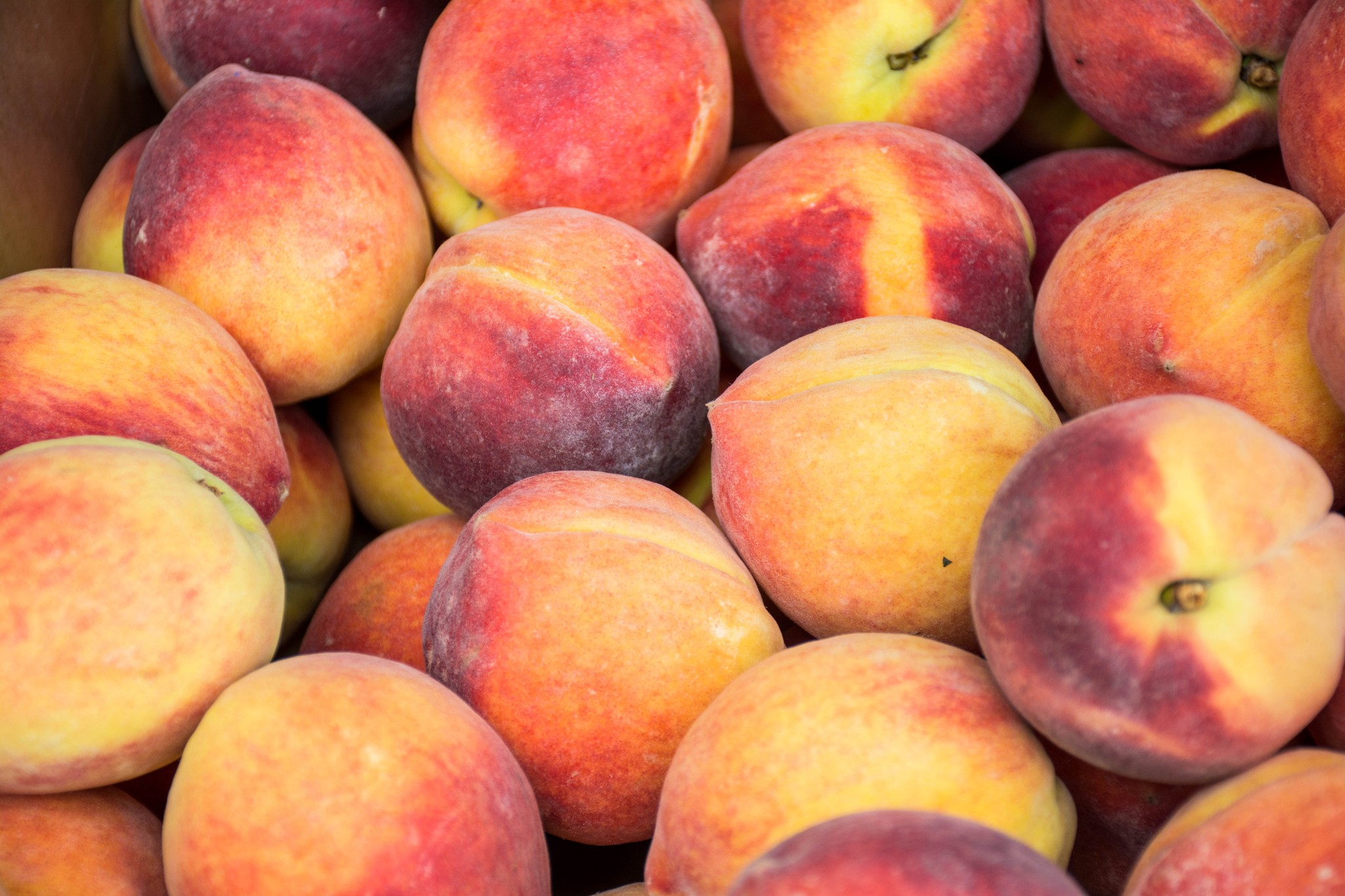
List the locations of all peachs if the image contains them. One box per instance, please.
[0,0,1345,896]
